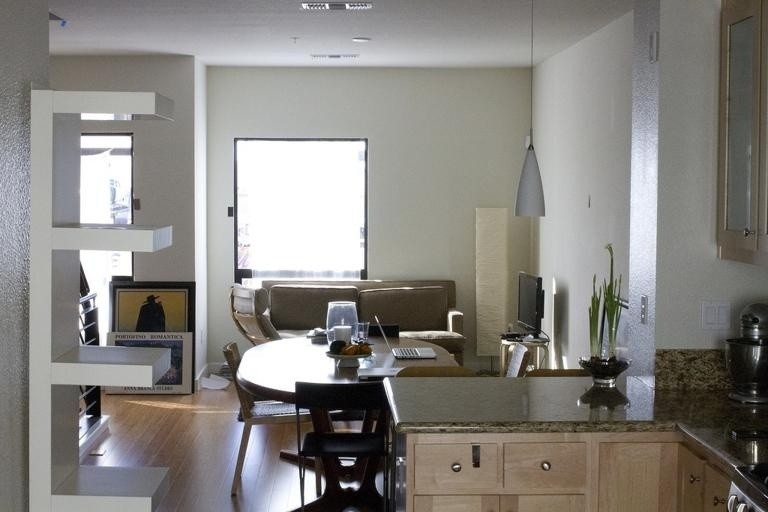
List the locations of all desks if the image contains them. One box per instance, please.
[498,338,550,376]
[236,334,458,495]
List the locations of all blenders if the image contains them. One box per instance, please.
[724,300,768,402]
[726,403,768,467]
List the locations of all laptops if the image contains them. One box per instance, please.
[375,315,438,360]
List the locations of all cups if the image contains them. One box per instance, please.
[356,321,371,340]
[326,301,360,348]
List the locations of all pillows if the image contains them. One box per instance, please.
[358,286,447,331]
[270,285,359,330]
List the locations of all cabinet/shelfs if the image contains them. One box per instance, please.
[598,437,679,512]
[27,89,173,512]
[679,443,731,512]
[716,0,768,266]
[411,436,588,511]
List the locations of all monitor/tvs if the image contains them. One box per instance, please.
[517,272,545,335]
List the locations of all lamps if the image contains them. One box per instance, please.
[515,0,546,217]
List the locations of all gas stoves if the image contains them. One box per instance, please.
[722,461,768,512]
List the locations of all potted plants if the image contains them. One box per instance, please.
[576,242,631,388]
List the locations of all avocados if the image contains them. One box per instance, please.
[330,340,346,354]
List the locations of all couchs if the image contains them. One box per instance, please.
[261,280,467,365]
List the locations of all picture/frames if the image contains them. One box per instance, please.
[107,331,193,394]
[109,281,195,395]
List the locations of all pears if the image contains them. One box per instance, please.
[342,344,371,355]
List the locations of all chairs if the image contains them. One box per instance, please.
[293,380,397,511]
[370,366,476,502]
[223,341,326,497]
[228,284,281,346]
[503,344,530,377]
